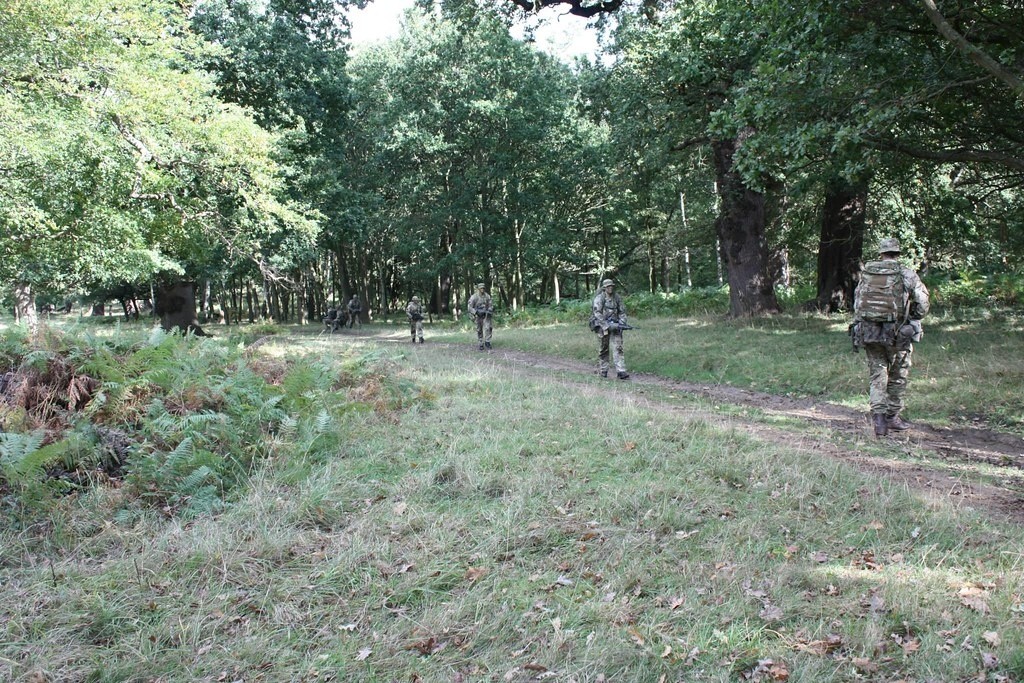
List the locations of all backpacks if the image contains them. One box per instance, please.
[857,262,905,323]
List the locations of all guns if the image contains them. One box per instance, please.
[413,314,424,321]
[475,306,492,320]
[608,318,634,335]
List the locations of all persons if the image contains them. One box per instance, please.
[322,294,363,332]
[592,278,630,380]
[405,296,424,344]
[467,283,494,350]
[847,238,930,440]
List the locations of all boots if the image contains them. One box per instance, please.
[885,414,912,431]
[872,415,889,438]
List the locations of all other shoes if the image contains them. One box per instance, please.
[485,342,491,349]
[480,346,484,350]
[412,338,415,342]
[419,338,424,343]
[617,372,629,379]
[601,371,608,377]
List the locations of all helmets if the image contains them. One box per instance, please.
[411,296,419,300]
[602,279,615,287]
[478,283,485,288]
[879,239,901,253]
[353,295,357,298]
